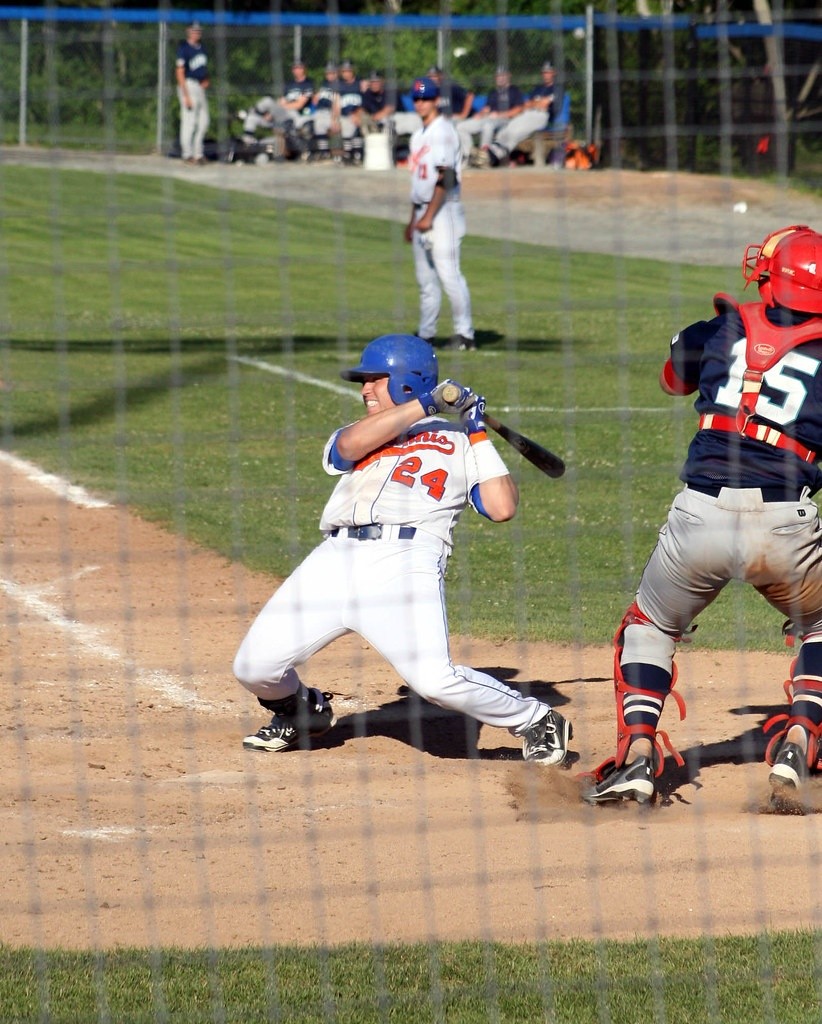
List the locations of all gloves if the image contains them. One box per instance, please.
[456,390,489,437]
[416,379,477,417]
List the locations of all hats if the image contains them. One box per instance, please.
[290,61,305,68]
[541,61,551,71]
[370,71,384,80]
[340,62,352,70]
[324,64,337,71]
[187,23,201,29]
[426,66,439,76]
[493,66,510,73]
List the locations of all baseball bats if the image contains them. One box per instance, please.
[442,384,566,479]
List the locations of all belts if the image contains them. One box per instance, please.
[686,482,801,503]
[414,202,427,208]
[331,524,415,540]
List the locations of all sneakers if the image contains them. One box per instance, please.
[767,742,808,805]
[578,755,654,806]
[241,688,336,751]
[520,710,573,768]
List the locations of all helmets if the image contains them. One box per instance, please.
[741,226,822,312]
[341,333,438,404]
[408,78,440,102]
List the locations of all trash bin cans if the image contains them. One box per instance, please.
[361,119,397,170]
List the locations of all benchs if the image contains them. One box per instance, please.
[264,88,574,169]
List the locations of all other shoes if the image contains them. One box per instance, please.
[443,335,477,355]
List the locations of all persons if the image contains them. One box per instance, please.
[176,22,208,163]
[572,227,822,815]
[404,76,476,352]
[233,335,571,766]
[227,62,564,170]
